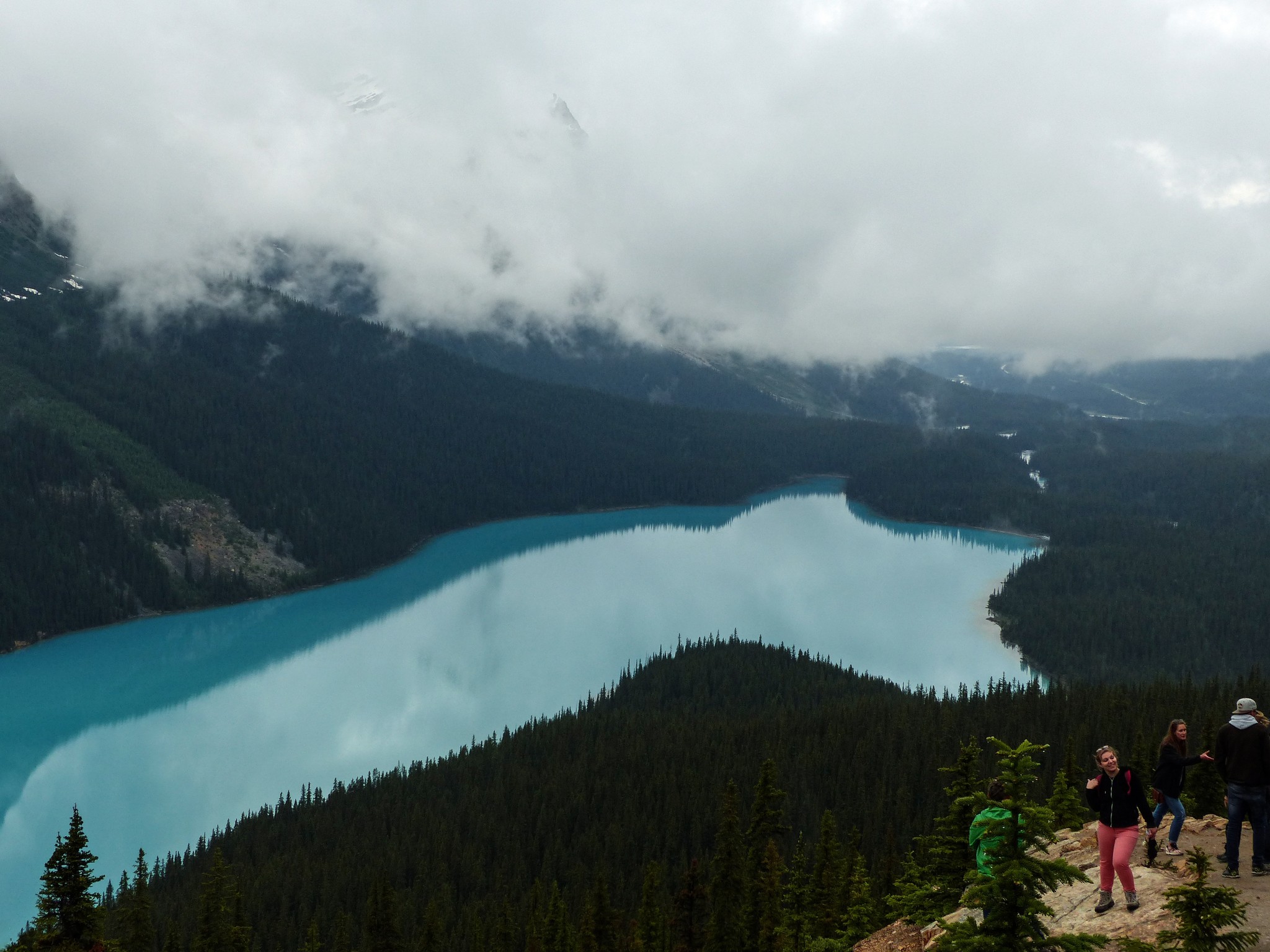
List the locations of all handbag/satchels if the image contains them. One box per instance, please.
[1152,788,1164,805]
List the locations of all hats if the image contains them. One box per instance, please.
[1232,698,1257,714]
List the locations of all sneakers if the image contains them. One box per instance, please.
[1251,860,1270,876]
[1124,889,1139,910]
[1222,865,1240,878]
[1216,852,1240,864]
[1143,838,1164,852]
[1166,843,1184,855]
[1094,888,1114,913]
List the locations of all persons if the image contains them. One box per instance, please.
[1086,745,1157,913]
[1216,711,1270,869]
[1142,719,1215,855]
[1214,698,1270,877]
[969,781,1025,921]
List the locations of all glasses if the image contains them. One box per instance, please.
[1096,745,1109,755]
